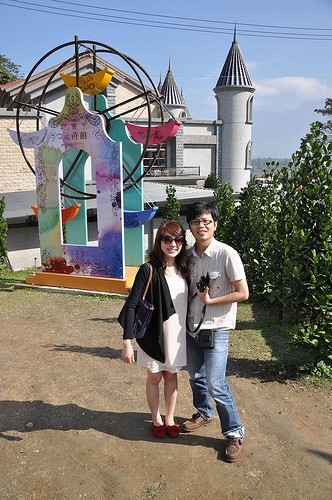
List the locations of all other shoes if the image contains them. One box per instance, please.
[152,420,166,438]
[163,417,179,437]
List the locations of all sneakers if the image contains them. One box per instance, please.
[183,412,212,432]
[223,426,245,463]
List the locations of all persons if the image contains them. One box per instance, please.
[121,221,191,439]
[184,201,249,462]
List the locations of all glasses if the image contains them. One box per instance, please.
[190,219,212,225]
[161,235,185,245]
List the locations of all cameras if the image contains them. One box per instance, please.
[196,271,219,293]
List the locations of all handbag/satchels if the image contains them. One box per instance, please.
[117,261,154,339]
